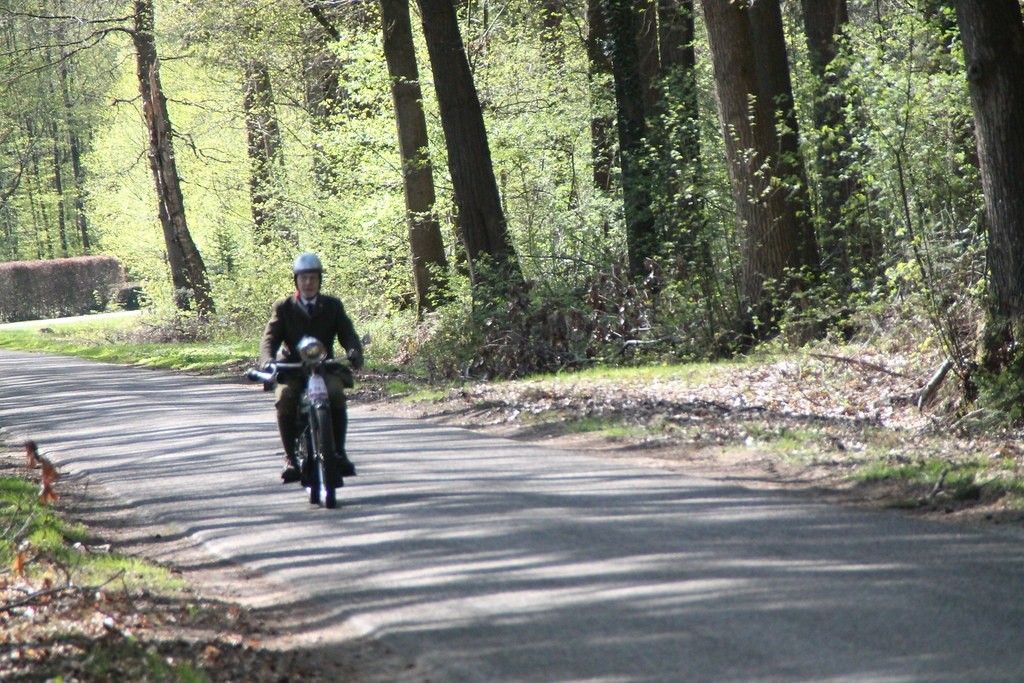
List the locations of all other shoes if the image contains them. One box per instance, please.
[336,453,356,476]
[281,458,300,478]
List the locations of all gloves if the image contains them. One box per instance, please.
[260,359,277,373]
[348,349,362,369]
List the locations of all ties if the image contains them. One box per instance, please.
[306,302,314,314]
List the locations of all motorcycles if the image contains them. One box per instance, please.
[246,333,372,509]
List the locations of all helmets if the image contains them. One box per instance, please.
[293,253,322,275]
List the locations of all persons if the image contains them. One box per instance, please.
[260,254,363,480]
